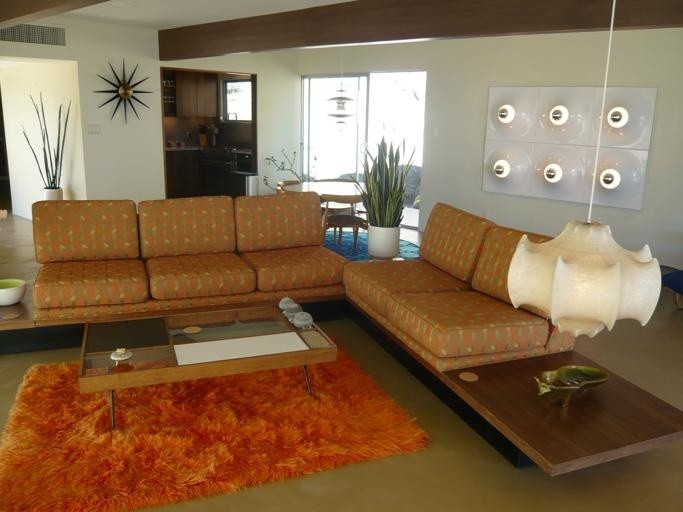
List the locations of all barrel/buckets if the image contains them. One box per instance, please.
[198,134,206,145]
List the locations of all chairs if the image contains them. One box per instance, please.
[274,178,367,255]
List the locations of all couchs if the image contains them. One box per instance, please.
[30,190,351,327]
[336,198,681,479]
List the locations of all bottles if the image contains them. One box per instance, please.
[278,296,312,329]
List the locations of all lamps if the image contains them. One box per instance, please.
[328,44,352,123]
[504,0,662,338]
[480,86,658,212]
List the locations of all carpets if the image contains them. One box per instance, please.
[1,334,431,512]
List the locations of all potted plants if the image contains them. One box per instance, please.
[353,134,416,257]
[21,87,70,200]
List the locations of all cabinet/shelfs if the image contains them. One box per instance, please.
[174,71,218,119]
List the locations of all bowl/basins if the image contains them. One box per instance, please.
[0,279,26,306]
